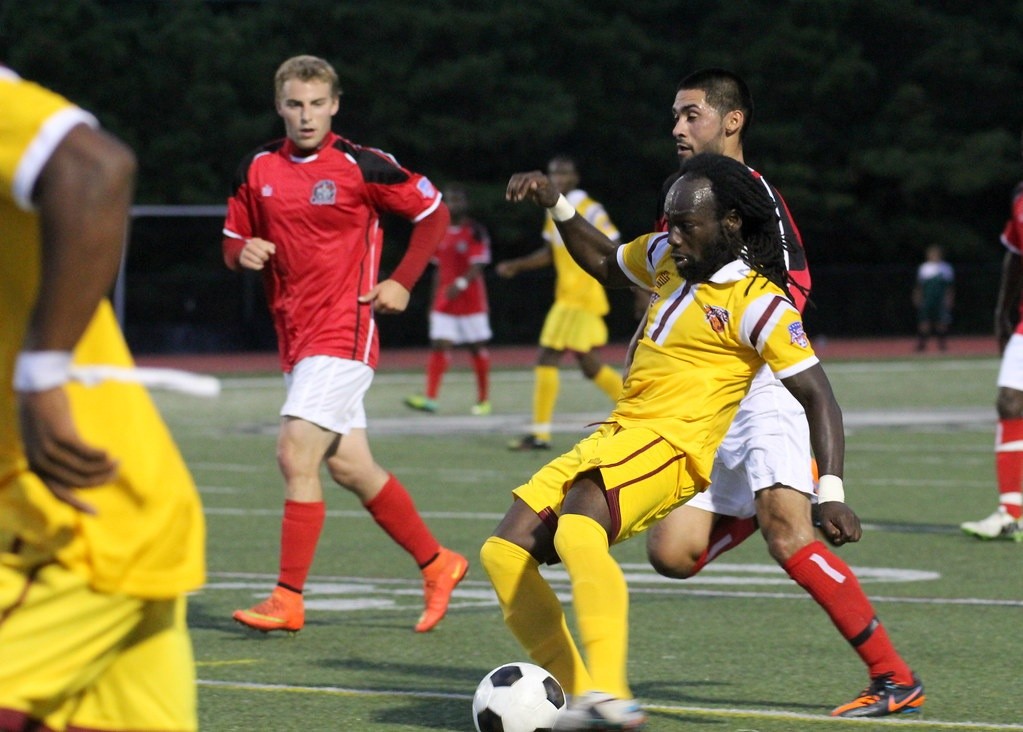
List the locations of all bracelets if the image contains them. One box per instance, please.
[455,277,468,290]
[11,349,71,392]
[818,474,845,505]
[549,193,575,221]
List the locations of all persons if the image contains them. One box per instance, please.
[913,246,955,351]
[222,58,469,633]
[647,69,925,718]
[0,298,208,732]
[0,64,135,515]
[402,188,493,416]
[960,189,1023,540]
[480,147,862,732]
[497,153,622,449]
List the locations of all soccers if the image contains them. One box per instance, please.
[472,662,567,731]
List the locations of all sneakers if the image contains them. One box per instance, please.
[555,693,645,730]
[831,673,925,718]
[405,394,438,411]
[472,402,490,415]
[508,436,549,450]
[234,595,304,633]
[959,507,1022,542]
[414,550,468,631]
